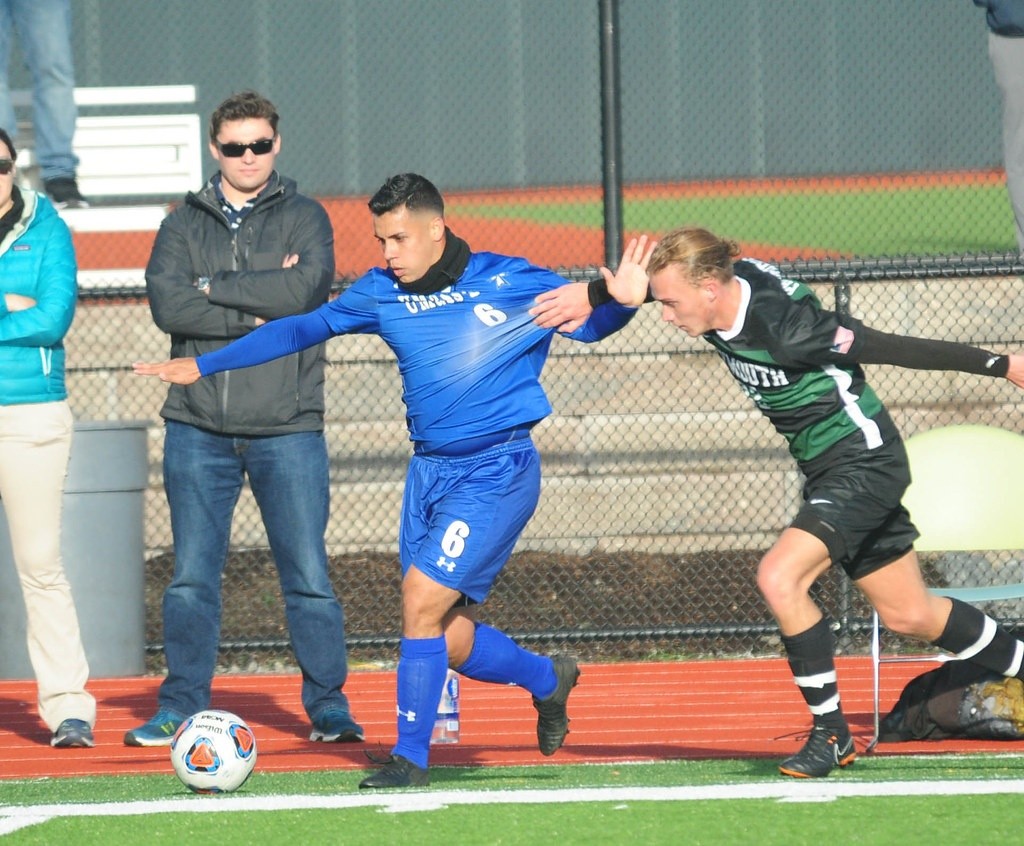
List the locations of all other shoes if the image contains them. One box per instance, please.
[46,177,89,210]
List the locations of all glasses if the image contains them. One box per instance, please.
[0,159,13,174]
[215,136,275,157]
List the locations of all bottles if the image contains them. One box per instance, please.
[430,669,460,745]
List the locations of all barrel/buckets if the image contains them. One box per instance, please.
[0,421,155,678]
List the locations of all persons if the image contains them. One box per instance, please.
[0,1,93,211]
[531,228,1023,779]
[133,175,659,791]
[971,1,1024,255]
[0,129,96,748]
[124,95,367,748]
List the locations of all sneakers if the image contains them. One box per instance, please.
[124,706,189,748]
[773,719,856,777]
[530,654,580,756]
[51,719,94,747]
[358,741,431,789]
[310,711,365,742]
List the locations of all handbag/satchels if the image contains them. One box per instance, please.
[879,662,1024,742]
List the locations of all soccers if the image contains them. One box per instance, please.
[169,710,257,794]
